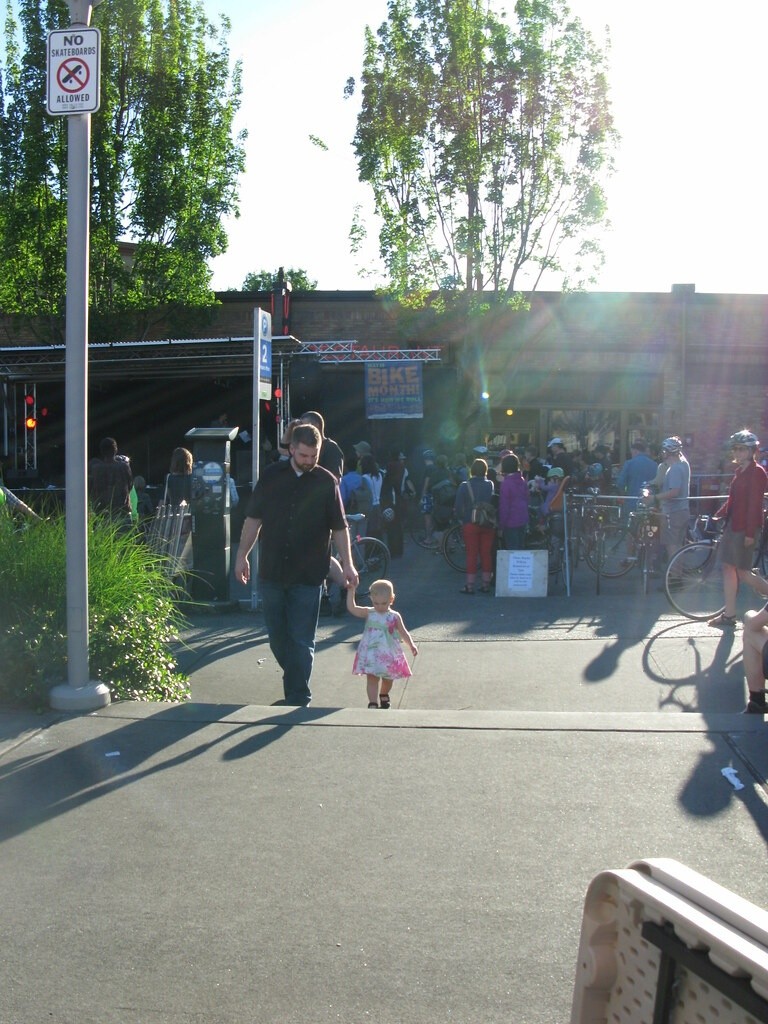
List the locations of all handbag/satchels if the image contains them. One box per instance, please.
[380,503,398,522]
[429,504,450,535]
[431,479,457,505]
[403,488,413,499]
[472,501,498,530]
[347,478,371,519]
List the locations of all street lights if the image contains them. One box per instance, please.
[271,264,293,458]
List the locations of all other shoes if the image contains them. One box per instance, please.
[739,701,768,714]
[478,586,490,594]
[657,577,685,593]
[379,693,390,709]
[536,524,547,532]
[620,554,638,567]
[368,702,378,709]
[459,585,474,594]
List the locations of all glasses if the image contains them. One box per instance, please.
[731,445,747,452]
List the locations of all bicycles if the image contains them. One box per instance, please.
[664,515,768,619]
[409,507,590,576]
[585,485,723,578]
[322,513,391,603]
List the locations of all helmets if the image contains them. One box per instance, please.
[473,446,488,456]
[588,463,603,477]
[728,430,760,447]
[661,436,683,454]
[497,449,514,458]
[547,467,565,480]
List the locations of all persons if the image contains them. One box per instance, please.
[269,441,416,572]
[0,486,43,524]
[740,602,768,714]
[88,437,239,602]
[707,429,768,626]
[235,411,418,709]
[418,437,612,594]
[616,436,690,593]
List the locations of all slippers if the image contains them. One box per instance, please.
[417,535,432,545]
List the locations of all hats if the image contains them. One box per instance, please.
[422,447,434,459]
[399,452,407,460]
[547,437,563,448]
[352,440,372,457]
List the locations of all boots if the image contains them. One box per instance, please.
[318,592,334,616]
[335,589,357,617]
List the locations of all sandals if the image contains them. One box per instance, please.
[706,612,737,625]
